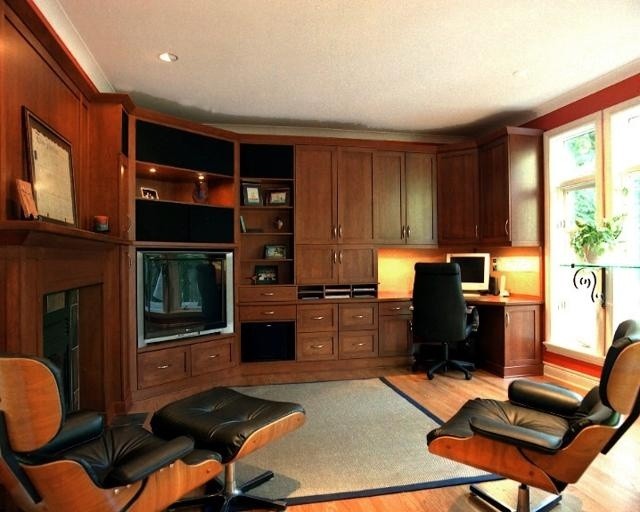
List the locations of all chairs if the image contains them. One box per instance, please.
[412,262,480,379]
[0,352,226,512]
[426,319,640,512]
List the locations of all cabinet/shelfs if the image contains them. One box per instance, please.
[0,0,545,429]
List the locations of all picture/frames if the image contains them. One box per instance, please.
[255,265,279,284]
[265,187,291,205]
[241,182,264,206]
[21,105,79,228]
[140,186,160,200]
[264,244,287,260]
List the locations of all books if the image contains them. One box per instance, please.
[14,177,40,220]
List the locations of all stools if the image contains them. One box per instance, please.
[149,386,305,512]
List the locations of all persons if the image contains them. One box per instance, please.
[256,270,274,280]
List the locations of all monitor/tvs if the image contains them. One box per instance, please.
[136,251,234,349]
[446,253,491,297]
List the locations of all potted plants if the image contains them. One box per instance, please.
[567,210,628,263]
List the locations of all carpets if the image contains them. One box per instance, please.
[143,377,510,505]
